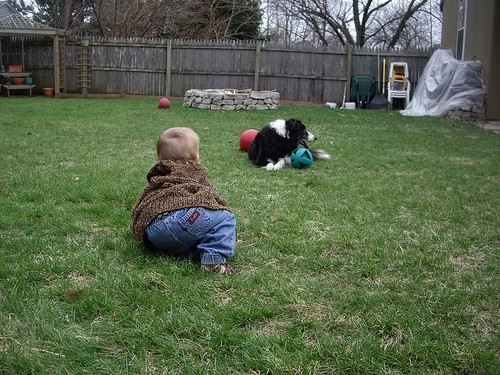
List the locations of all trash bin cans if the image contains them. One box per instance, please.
[351,74,375,108]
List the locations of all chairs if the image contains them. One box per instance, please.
[387,62,411,110]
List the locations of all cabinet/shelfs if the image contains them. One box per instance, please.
[0,73,37,97]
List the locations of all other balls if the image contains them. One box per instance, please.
[239,128,259,150]
[159,97,171,108]
[290,147,314,169]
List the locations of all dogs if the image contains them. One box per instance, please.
[248,118,331,171]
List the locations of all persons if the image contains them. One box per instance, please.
[131,127,238,275]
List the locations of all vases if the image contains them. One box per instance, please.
[42,88,54,97]
[26,78,32,84]
[12,78,25,85]
[9,66,22,73]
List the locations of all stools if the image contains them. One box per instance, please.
[392,75,406,90]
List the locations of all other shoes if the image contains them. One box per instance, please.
[200,263,236,274]
